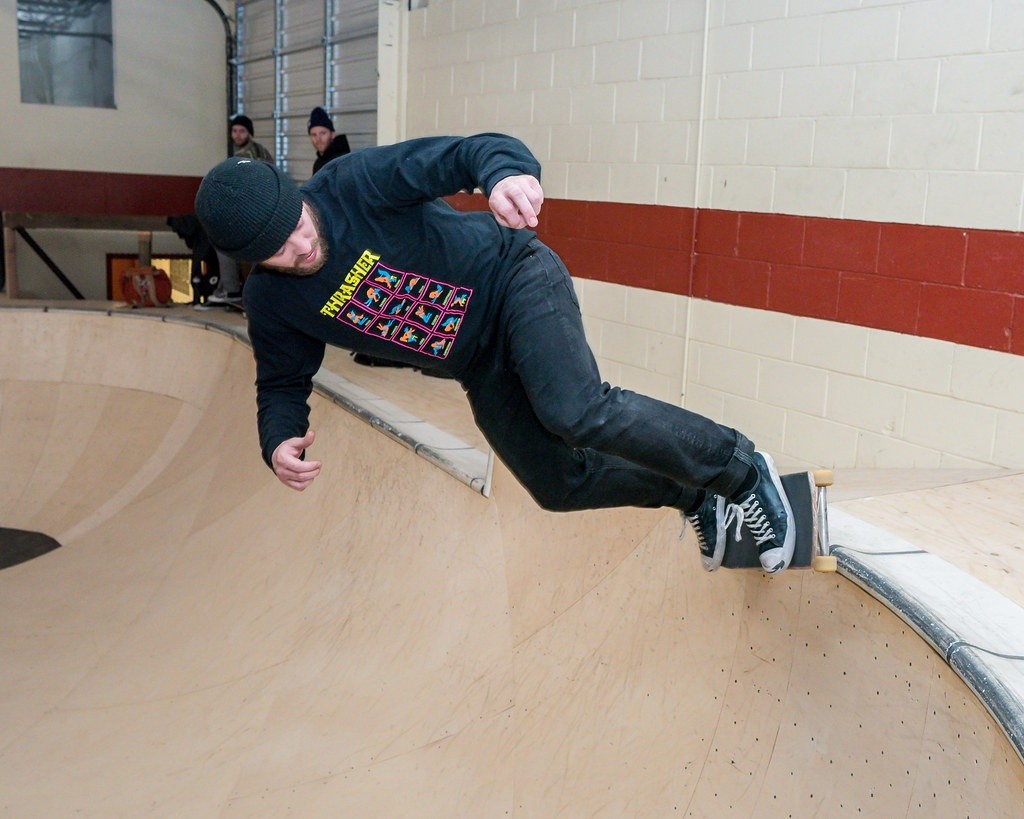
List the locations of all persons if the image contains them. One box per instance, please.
[194,132,795,572]
[194,116,273,310]
[307,106,351,176]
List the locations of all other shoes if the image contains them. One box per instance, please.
[208,289,243,302]
[194,299,227,311]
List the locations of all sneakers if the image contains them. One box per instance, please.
[720,452,795,575]
[681,493,727,572]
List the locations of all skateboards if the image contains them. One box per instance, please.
[720,471,838,571]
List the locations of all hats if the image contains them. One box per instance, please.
[195,156,302,264]
[231,116,254,138]
[307,107,335,134]
